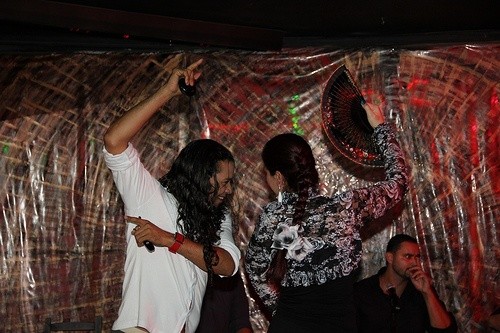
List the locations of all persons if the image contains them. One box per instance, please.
[102,58,241,333]
[243,102,408,333]
[357,234,458,333]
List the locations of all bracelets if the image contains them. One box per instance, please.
[169,232,184,254]
